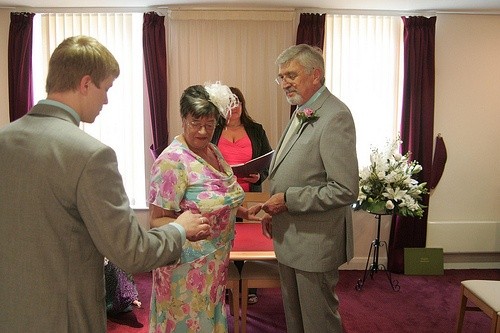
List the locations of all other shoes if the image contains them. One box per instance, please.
[247,293,258,304]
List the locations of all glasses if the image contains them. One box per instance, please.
[274,71,305,85]
[184,117,219,130]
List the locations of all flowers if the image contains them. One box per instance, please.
[353,130,431,221]
[295,107,320,135]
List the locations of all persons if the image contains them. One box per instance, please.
[261,43,359,333]
[149,84,265,332]
[211,86,274,304]
[0,35,211,332]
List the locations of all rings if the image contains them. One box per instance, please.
[200,217,204,223]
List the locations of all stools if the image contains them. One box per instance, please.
[225,261,281,333]
[455,279,500,333]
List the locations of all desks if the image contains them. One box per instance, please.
[229,222,276,260]
